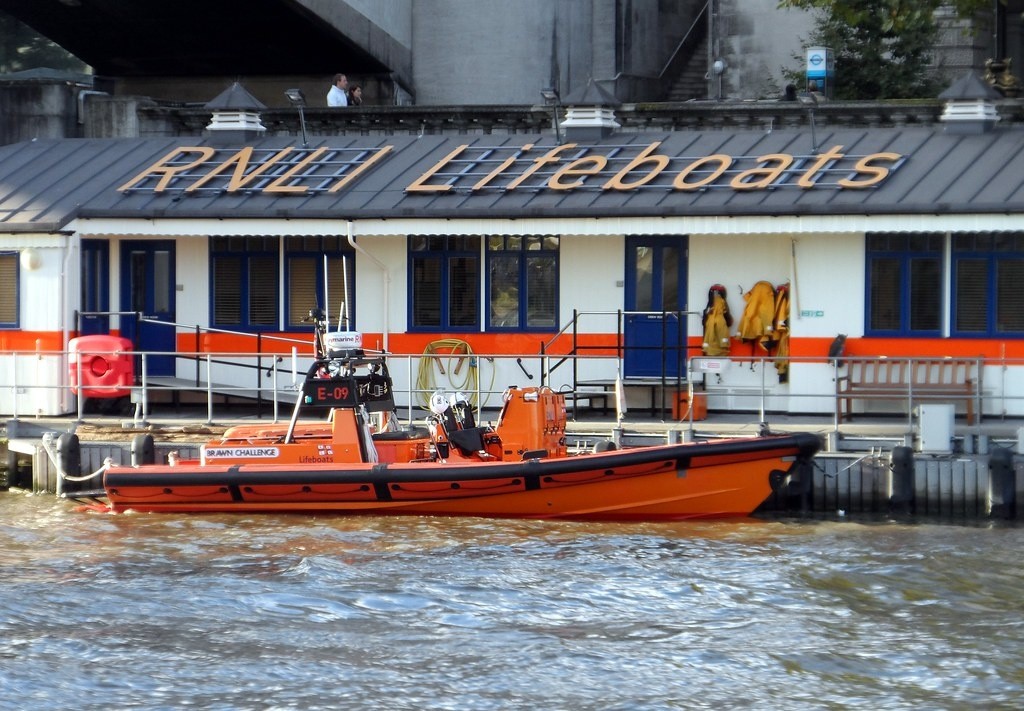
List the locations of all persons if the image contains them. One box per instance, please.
[326,74,348,106]
[345,84,362,106]
[803,80,829,102]
[779,84,798,101]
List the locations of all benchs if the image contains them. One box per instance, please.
[837,354,977,425]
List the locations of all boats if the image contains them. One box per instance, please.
[104,249,827,524]
